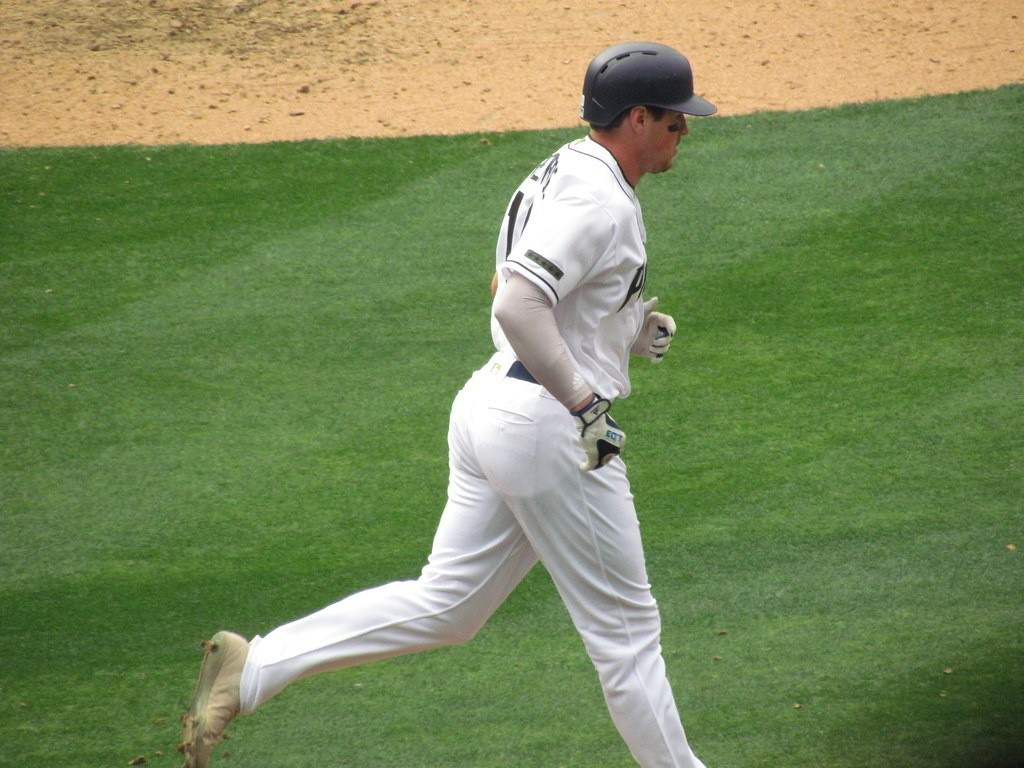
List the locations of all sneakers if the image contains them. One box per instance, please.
[176,630,249,768]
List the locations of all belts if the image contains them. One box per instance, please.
[506,361,542,386]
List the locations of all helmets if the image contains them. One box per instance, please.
[579,41,717,127]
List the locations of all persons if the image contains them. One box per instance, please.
[183,41,718,768]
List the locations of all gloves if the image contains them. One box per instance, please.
[630,297,676,363]
[570,394,625,471]
